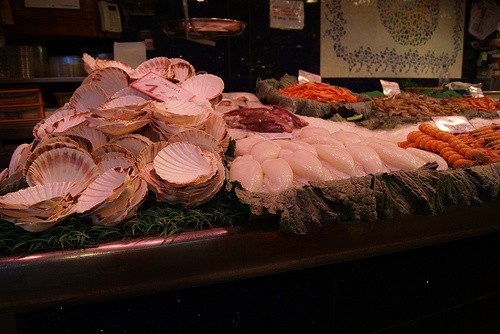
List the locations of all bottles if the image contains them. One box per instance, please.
[438,65,450,87]
[476,52,490,92]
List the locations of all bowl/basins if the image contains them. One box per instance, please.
[54,92,74,107]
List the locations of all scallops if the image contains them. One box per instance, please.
[0,55,232,233]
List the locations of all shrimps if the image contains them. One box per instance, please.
[397,123,500,170]
[372,90,500,119]
[279,81,358,105]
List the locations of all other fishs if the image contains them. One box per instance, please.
[222,105,310,134]
